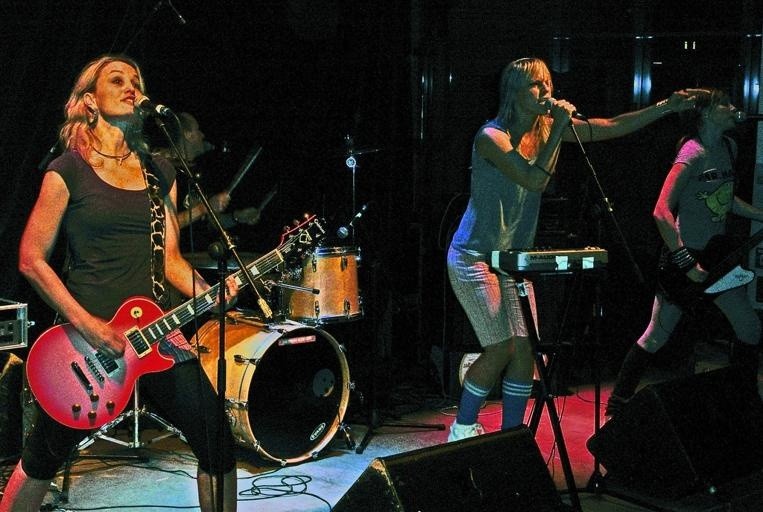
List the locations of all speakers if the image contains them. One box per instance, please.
[585,363,763,503]
[0,349,25,468]
[329,423,567,512]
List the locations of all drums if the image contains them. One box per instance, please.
[274,242,364,328]
[186,309,351,465]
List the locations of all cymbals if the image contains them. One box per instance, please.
[180,250,262,271]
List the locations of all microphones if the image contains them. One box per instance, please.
[734,111,763,123]
[545,98,589,122]
[134,94,171,117]
[336,202,371,239]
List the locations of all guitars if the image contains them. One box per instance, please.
[26,214,327,431]
[658,229,763,318]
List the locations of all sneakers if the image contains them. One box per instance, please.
[448,419,485,443]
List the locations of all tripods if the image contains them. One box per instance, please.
[556,288,676,512]
[76,376,188,452]
[356,259,446,454]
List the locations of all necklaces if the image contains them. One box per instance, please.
[91,146,134,166]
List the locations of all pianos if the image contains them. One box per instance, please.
[486,244,608,274]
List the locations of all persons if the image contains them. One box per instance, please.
[603,89,762,420]
[148,110,264,313]
[446,57,711,443]
[0,54,240,512]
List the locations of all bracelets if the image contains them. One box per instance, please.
[670,244,696,276]
[199,203,205,214]
[230,210,240,225]
[655,98,672,117]
[535,164,554,179]
